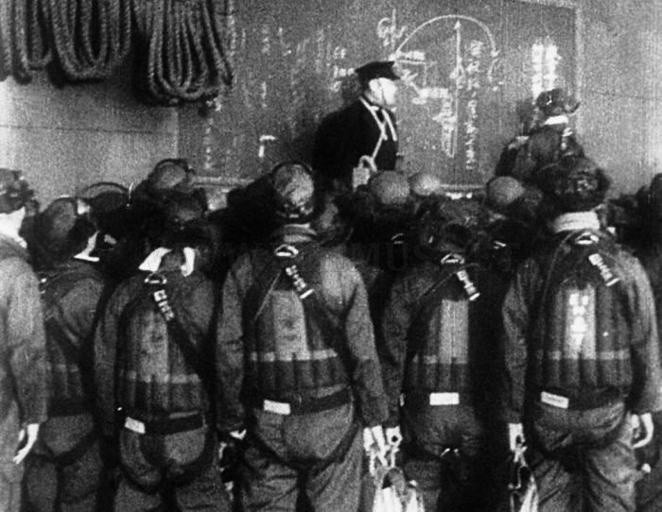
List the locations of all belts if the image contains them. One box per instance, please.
[45,399,90,417]
[408,390,476,408]
[119,413,205,435]
[247,390,351,414]
[528,387,623,411]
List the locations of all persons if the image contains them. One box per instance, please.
[508,89,584,187]
[1,157,662,512]
[313,60,401,201]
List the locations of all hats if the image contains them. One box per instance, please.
[366,169,411,210]
[410,170,448,200]
[145,157,192,195]
[412,196,496,270]
[535,152,612,212]
[0,168,31,214]
[355,61,400,84]
[485,174,528,213]
[164,193,204,226]
[35,197,101,260]
[262,159,326,225]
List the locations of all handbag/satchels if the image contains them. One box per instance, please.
[501,457,539,511]
[361,464,425,512]
